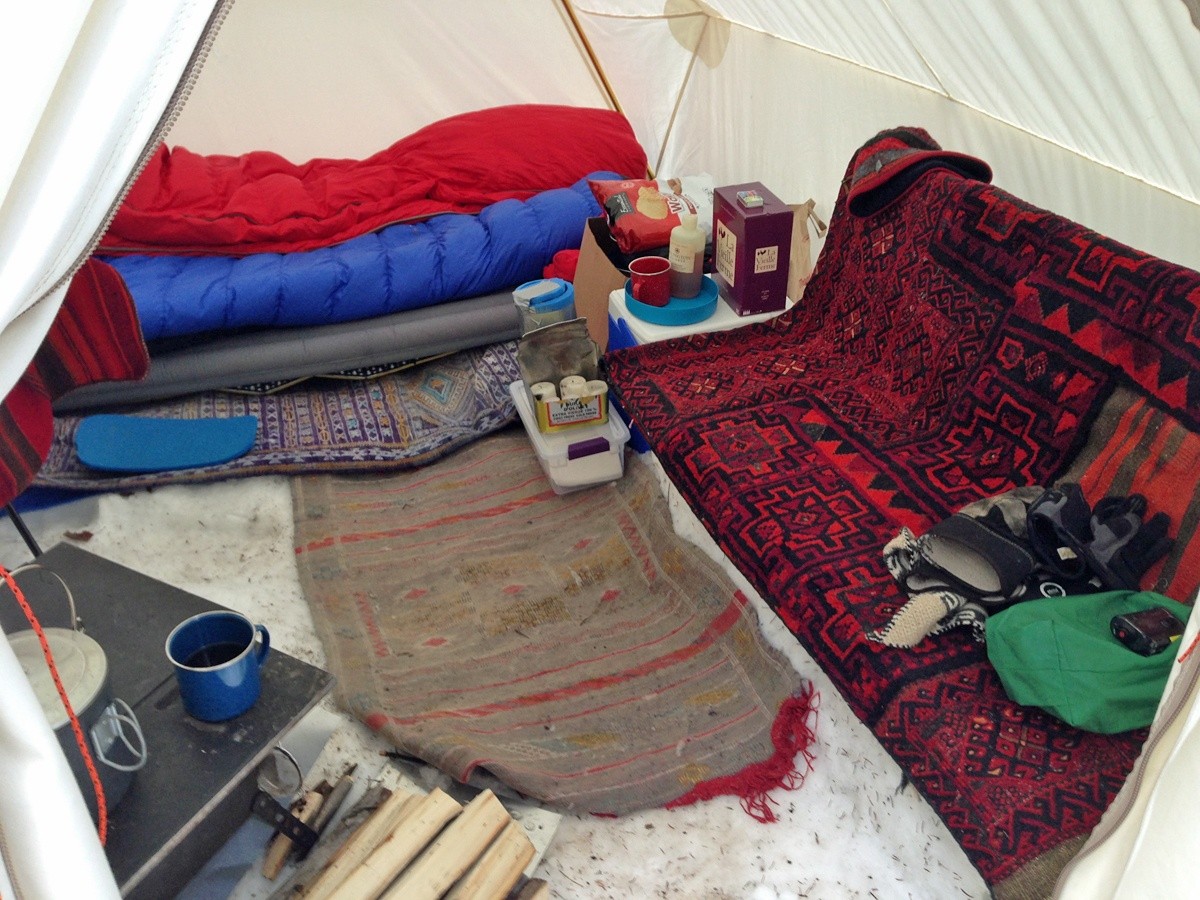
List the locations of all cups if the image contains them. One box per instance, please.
[628,256,672,307]
[165,611,270,721]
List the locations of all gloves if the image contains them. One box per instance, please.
[918,489,1178,602]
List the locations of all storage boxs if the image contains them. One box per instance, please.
[712,181,794,318]
[518,316,609,431]
[510,381,631,494]
[573,214,629,354]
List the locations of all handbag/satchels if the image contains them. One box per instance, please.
[982,588,1193,734]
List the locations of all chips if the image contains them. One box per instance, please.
[636,186,669,219]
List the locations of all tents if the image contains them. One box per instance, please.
[0,0,1200,900]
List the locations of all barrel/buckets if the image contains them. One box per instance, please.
[0,564,147,823]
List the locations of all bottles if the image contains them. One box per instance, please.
[669,215,706,298]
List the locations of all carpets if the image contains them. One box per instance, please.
[17,332,519,486]
[278,432,814,815]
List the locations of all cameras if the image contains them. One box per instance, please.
[1110,606,1186,655]
